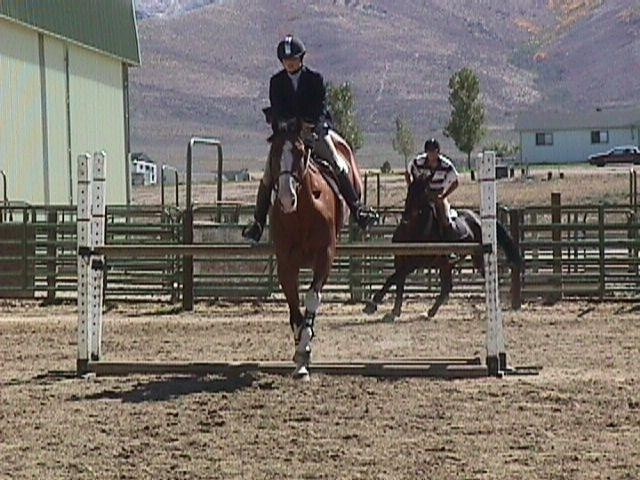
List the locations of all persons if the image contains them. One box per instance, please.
[242,32,380,245]
[403,138,463,242]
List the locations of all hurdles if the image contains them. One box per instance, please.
[76,150,511,378]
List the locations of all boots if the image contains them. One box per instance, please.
[336,169,373,223]
[245,179,272,243]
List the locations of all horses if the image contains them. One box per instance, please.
[361,164,526,324]
[264,113,351,381]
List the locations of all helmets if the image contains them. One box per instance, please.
[277,34,306,59]
[425,139,440,155]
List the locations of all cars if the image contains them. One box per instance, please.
[587,145,640,167]
